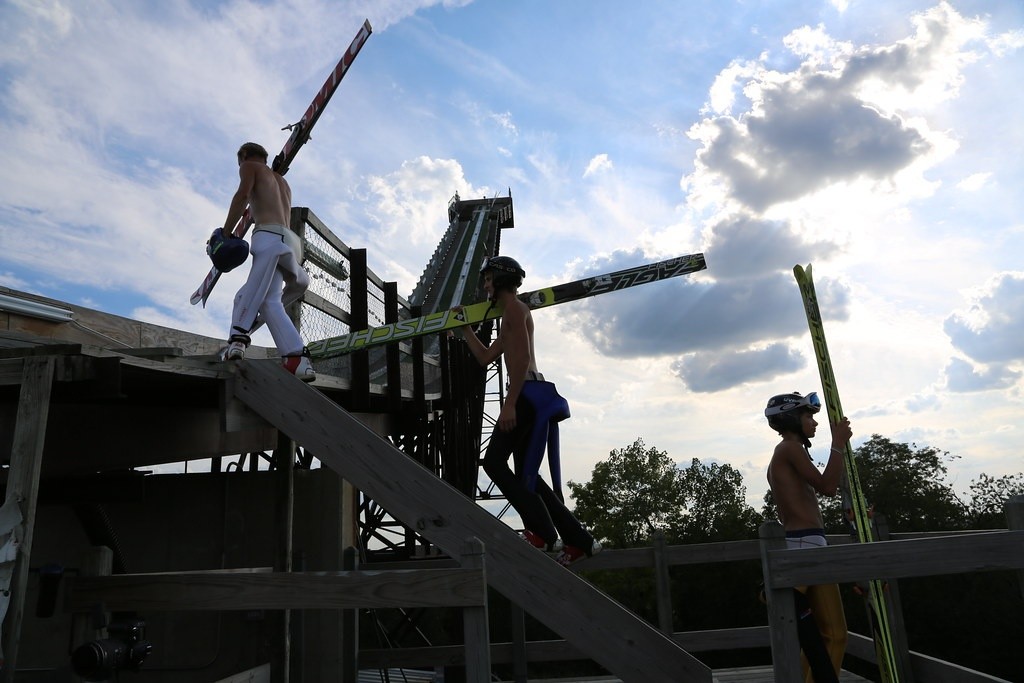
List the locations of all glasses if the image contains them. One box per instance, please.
[803,391,820,413]
[479,255,490,273]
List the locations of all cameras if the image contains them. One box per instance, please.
[70,617,152,681]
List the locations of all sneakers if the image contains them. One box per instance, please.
[219,341,245,361]
[280,356,315,382]
[519,529,558,551]
[554,540,601,565]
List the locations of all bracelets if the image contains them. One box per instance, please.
[831,447,842,455]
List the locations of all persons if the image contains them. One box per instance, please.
[764,393,849,683]
[221,143,317,384]
[450,256,604,569]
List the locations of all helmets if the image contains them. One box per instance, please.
[766,391,814,431]
[206,227,248,272]
[479,255,524,289]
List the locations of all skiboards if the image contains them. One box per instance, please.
[187,15,377,309]
[791,260,900,682]
[305,252,709,356]
